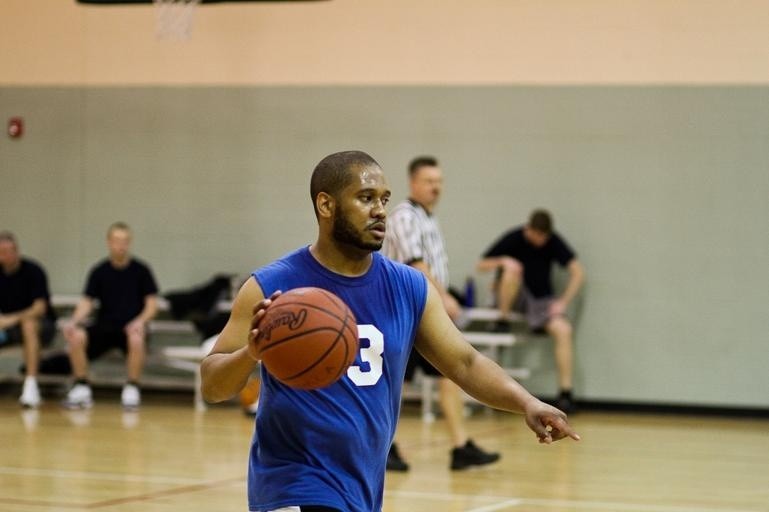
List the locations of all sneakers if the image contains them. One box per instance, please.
[21,382,140,409]
[450,437,498,470]
[387,443,407,471]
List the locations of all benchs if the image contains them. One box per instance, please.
[0,298,525,420]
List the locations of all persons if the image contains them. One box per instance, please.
[477,205,583,416]
[0,230,55,408]
[202,150,582,510]
[62,220,160,407]
[375,154,506,475]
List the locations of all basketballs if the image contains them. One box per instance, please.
[258,287,359,390]
